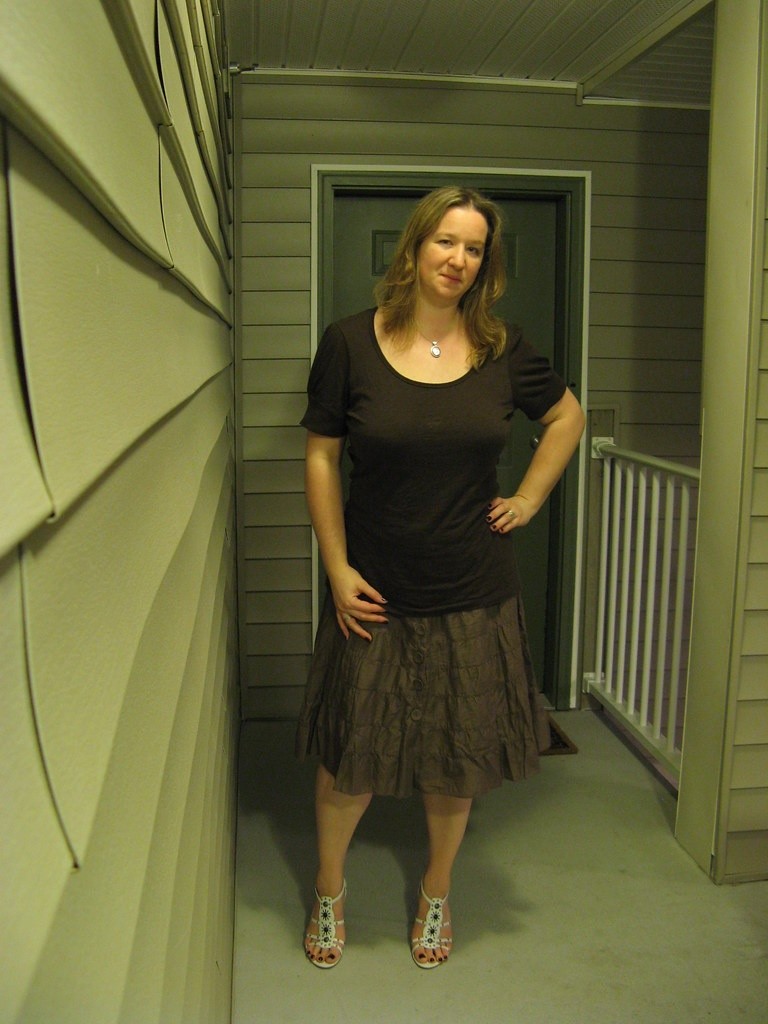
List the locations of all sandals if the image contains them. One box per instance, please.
[411,872,452,969]
[305,877,347,969]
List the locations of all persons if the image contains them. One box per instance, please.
[302,186,583,971]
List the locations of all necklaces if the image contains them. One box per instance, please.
[413,321,451,358]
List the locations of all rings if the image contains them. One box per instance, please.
[508,511,515,518]
[343,615,352,623]
